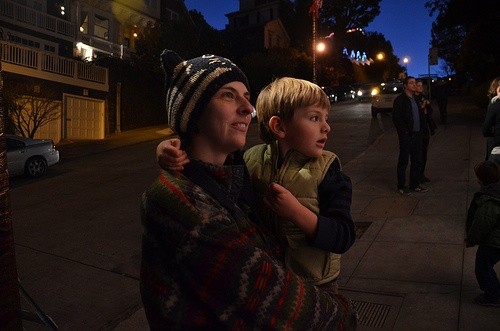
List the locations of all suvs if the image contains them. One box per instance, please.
[371,83,403,118]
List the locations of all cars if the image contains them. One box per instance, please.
[0,134,59,178]
[321,82,379,103]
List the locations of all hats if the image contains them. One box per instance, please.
[159,48,250,139]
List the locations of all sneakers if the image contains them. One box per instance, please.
[474,291,500,307]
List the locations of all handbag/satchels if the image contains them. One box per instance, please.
[463,191,500,255]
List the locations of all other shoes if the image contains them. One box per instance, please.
[409,185,431,192]
[399,185,413,196]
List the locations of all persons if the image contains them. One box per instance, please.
[463,75,500,306]
[393,76,428,195]
[155,77,354,294]
[412,79,437,182]
[137,50,354,331]
[437,78,448,123]
